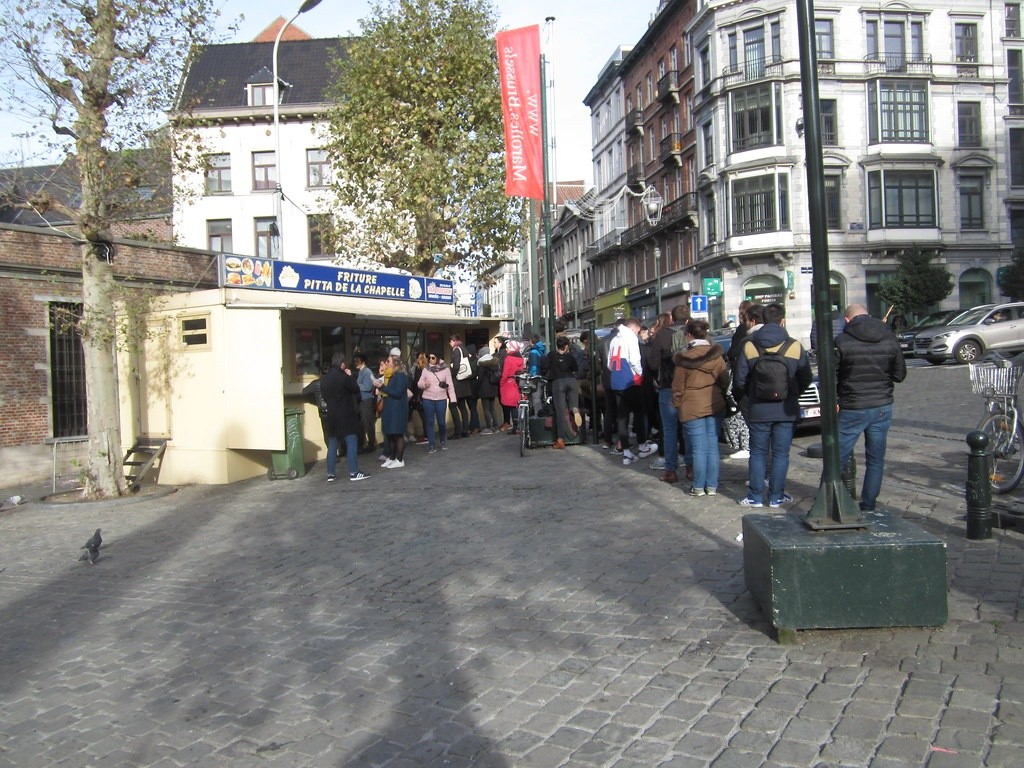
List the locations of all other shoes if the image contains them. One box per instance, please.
[553,439,565,450]
[687,469,695,480]
[745,479,770,488]
[659,471,678,483]
[573,407,583,427]
[859,501,876,514]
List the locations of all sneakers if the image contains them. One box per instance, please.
[326,420,520,481]
[603,428,686,470]
[770,494,793,508]
[739,497,763,508]
[689,486,706,497]
[705,486,717,495]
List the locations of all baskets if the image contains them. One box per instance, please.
[968,360,1022,397]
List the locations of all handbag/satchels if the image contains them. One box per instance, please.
[410,395,422,409]
[450,347,472,380]
[724,395,740,418]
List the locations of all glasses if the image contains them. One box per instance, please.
[429,357,436,360]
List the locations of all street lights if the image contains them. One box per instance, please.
[654,246,662,316]
[271,0,325,263]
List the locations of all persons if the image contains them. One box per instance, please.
[992,311,1003,321]
[300,332,546,481]
[542,304,730,496]
[722,300,812,508]
[832,305,907,512]
[810,319,816,350]
[831,310,846,339]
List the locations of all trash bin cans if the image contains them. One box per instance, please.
[267,408,305,481]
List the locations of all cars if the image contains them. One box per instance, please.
[914,302,1024,365]
[707,329,841,432]
[895,309,967,355]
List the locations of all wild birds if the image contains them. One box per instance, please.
[80,529,103,551]
[78,543,100,564]
[4,495,24,506]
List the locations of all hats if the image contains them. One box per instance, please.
[390,348,401,358]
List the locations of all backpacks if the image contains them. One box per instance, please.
[488,365,502,384]
[670,325,689,355]
[750,337,792,402]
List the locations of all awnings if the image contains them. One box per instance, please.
[296,307,515,361]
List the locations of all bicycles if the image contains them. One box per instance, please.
[967,355,1024,495]
[508,374,548,458]
